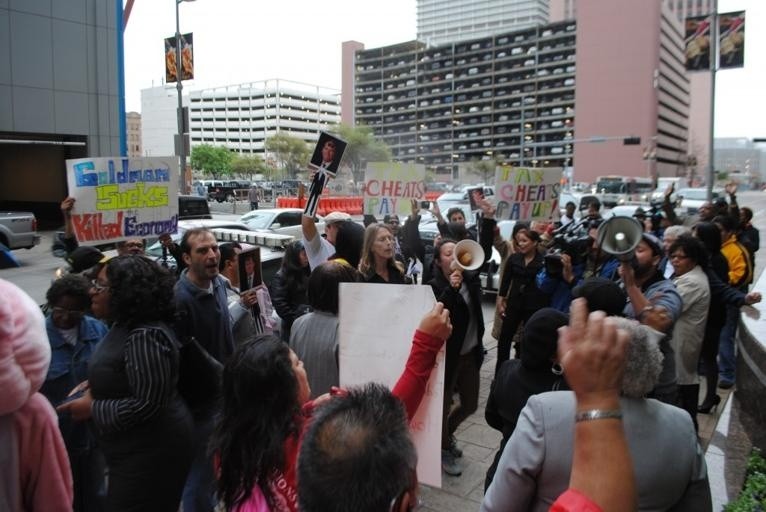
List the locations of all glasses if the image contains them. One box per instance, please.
[92,280,113,293]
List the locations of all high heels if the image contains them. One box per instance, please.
[698,395,721,414]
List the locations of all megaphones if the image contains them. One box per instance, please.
[449,238,486,288]
[594,215,644,268]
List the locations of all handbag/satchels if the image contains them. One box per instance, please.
[179,338,224,410]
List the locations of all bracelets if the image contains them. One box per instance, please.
[572,408,626,424]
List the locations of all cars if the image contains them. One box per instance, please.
[55,179,327,294]
[0,211,41,251]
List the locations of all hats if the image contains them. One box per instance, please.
[324,212,352,224]
[572,279,626,316]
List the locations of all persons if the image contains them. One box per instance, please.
[53,253,196,512]
[311,139,340,173]
[294,295,639,512]
[1,170,763,418]
[0,278,75,512]
[477,314,712,512]
[212,299,453,512]
[37,273,111,512]
[482,307,576,495]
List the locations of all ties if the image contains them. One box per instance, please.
[247,276,251,287]
[322,163,326,168]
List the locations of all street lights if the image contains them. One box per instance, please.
[176,0,197,197]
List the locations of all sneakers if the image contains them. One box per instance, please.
[443,424,464,476]
[719,380,733,389]
